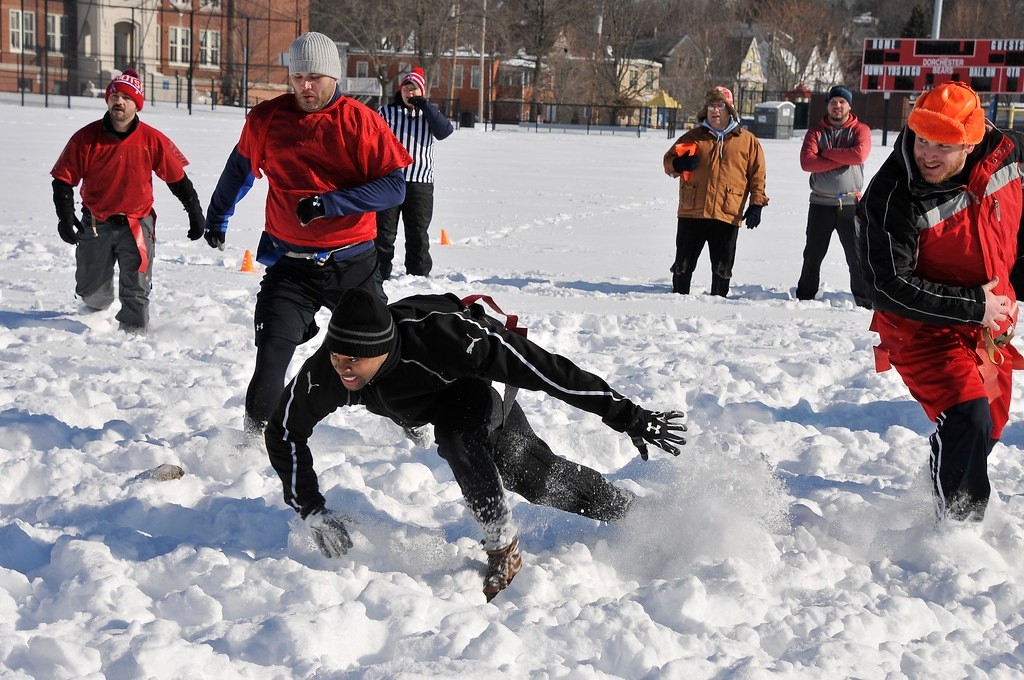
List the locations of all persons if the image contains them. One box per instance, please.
[377,67,453,282]
[854,81,1024,524]
[664,86,769,296]
[205,32,414,438]
[50,69,205,333]
[264,287,687,602]
[797,85,873,310]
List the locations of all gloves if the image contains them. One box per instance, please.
[187,204,205,241]
[626,406,687,462]
[203,228,225,249]
[673,150,700,174]
[297,195,325,228]
[406,96,428,109]
[742,206,763,230]
[55,201,84,245]
[304,504,354,559]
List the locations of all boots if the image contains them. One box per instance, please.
[711,273,730,299]
[672,274,692,294]
[482,536,522,603]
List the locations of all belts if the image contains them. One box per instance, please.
[82,206,128,230]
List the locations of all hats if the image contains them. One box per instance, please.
[326,288,396,359]
[697,87,739,124]
[908,81,986,145]
[106,70,145,114]
[827,85,852,107]
[400,68,426,97]
[289,32,342,81]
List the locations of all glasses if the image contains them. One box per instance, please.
[705,103,728,110]
